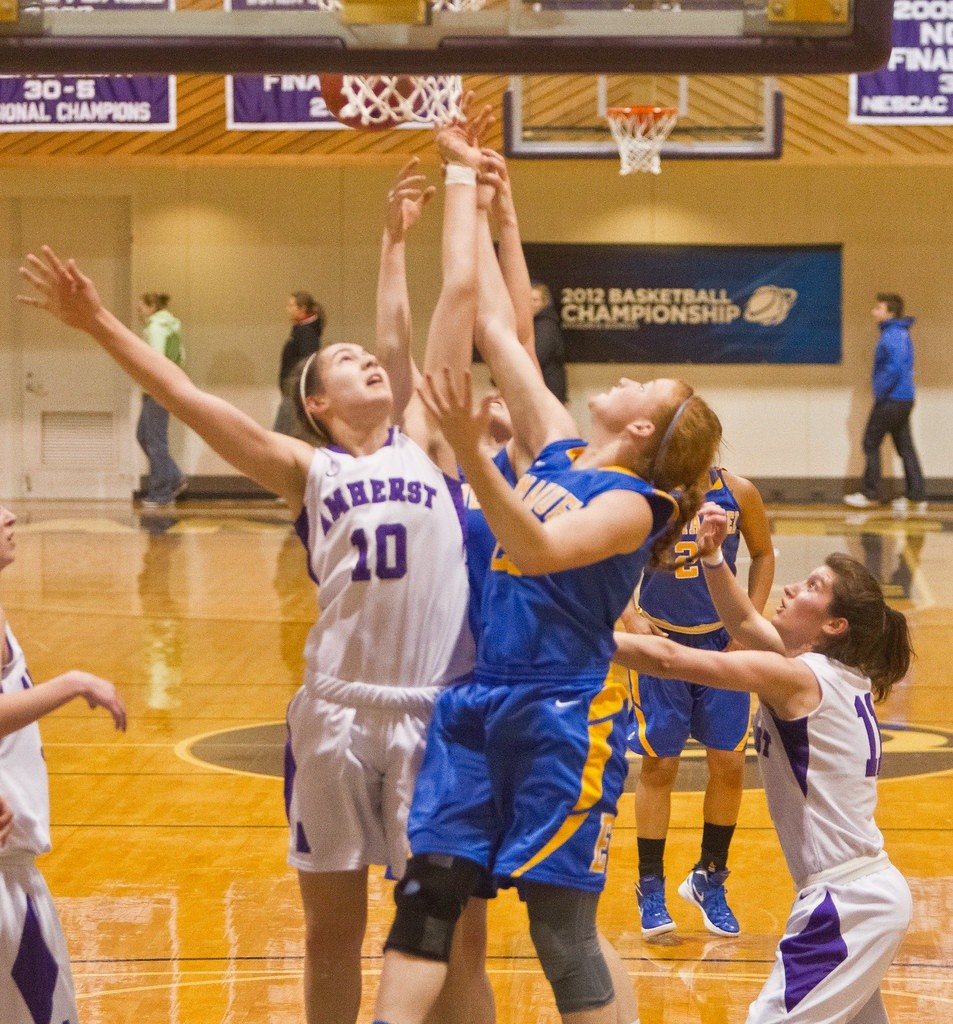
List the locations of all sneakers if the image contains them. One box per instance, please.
[842,492,880,507]
[140,494,175,507]
[635,877,677,944]
[676,866,743,938]
[155,477,189,504]
[893,497,927,510]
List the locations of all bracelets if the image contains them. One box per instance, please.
[702,546,723,568]
[445,164,478,186]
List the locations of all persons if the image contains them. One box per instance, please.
[0,504,127,1024]
[135,292,189,507]
[620,411,775,938]
[272,291,327,507]
[490,283,571,407]
[611,502,913,1024]
[843,292,929,509]
[16,87,722,1024]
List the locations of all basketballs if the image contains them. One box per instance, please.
[317,69,426,135]
